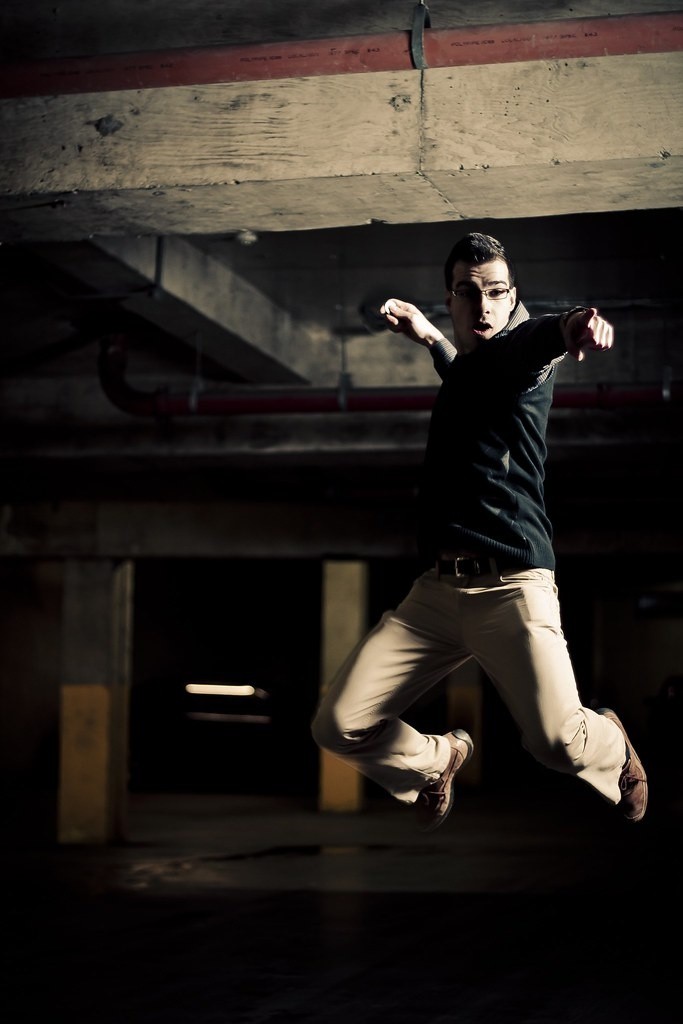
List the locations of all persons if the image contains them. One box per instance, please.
[313,232,649,821]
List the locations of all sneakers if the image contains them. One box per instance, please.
[595,708,648,823]
[415,729,474,831]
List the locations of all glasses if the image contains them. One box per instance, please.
[450,288,511,300]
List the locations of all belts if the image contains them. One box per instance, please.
[432,556,532,579]
